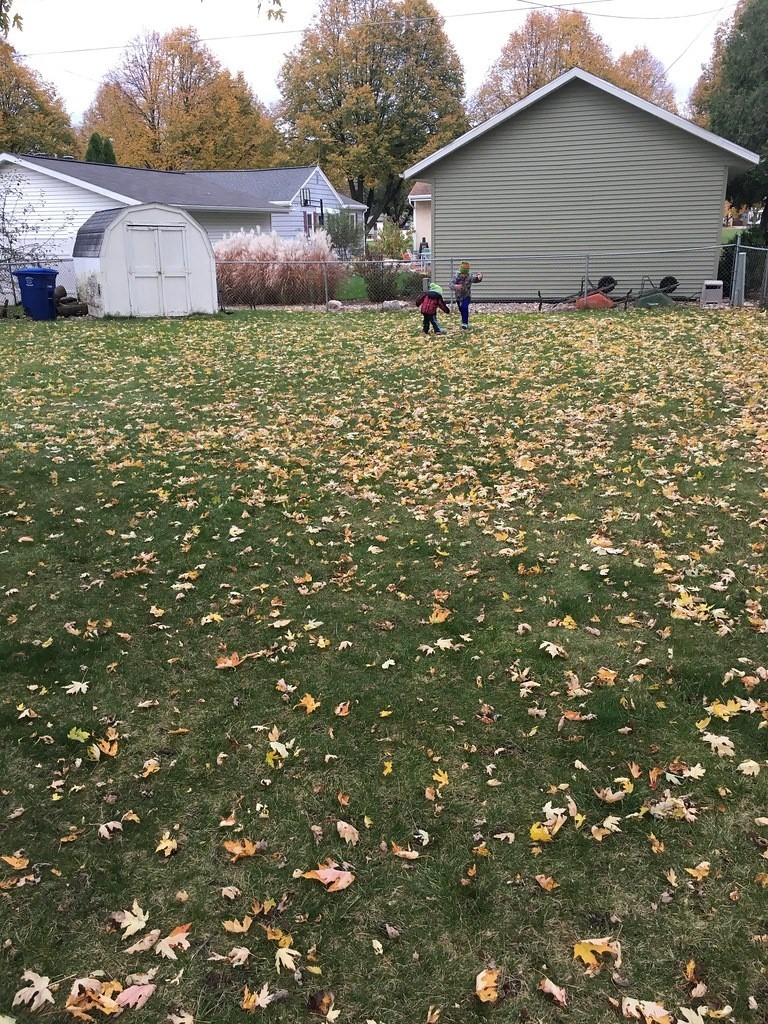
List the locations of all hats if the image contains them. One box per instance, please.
[459,260,470,274]
[430,283,442,293]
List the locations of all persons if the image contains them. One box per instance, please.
[416,282,449,334]
[449,260,484,329]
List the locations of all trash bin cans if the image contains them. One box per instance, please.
[11,269,60,321]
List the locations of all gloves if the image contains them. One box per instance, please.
[475,273,483,282]
[454,284,463,291]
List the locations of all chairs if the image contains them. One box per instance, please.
[407,248,417,259]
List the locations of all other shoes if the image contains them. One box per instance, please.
[462,323,467,330]
[436,332,441,334]
